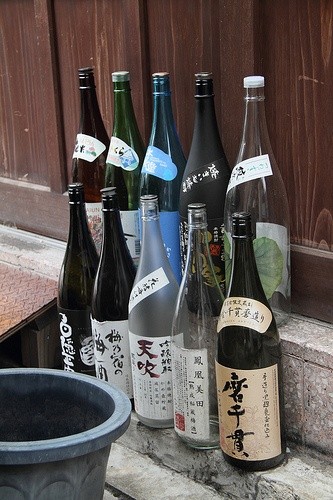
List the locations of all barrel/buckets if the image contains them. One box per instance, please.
[0,368,131,500]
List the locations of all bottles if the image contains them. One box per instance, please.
[105,71,147,267]
[172,203,225,450]
[129,195,179,429]
[179,72,232,316]
[57,182,99,377]
[71,67,110,256]
[216,212,286,473]
[90,186,137,410]
[225,76,290,328]
[138,73,187,285]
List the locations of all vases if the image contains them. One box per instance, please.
[0,368,133,500]
